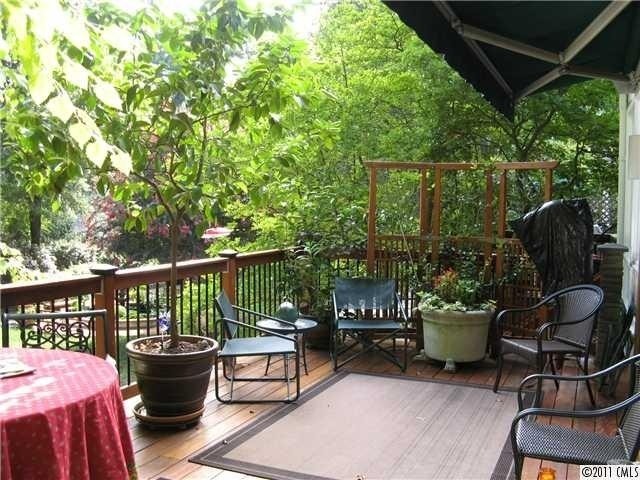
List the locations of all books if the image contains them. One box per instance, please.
[0,352,36,380]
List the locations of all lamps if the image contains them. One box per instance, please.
[628,135,640,179]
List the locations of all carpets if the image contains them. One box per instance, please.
[188,367,546,480]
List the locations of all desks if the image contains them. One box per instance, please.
[277,236,355,351]
[0,347,139,479]
[256,318,318,376]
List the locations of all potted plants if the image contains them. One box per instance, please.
[411,272,499,374]
[52,0,300,418]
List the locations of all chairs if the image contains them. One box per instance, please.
[329,276,408,373]
[214,289,300,404]
[2,309,110,358]
[493,284,605,393]
[511,353,640,480]
